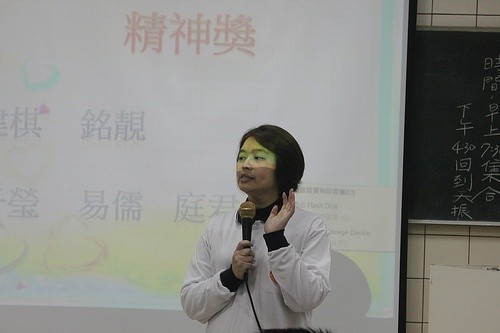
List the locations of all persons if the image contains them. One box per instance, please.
[180,125,330,333]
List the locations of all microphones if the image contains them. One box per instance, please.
[239,202,256,281]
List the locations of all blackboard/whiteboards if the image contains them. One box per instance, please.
[401,24,500,228]
